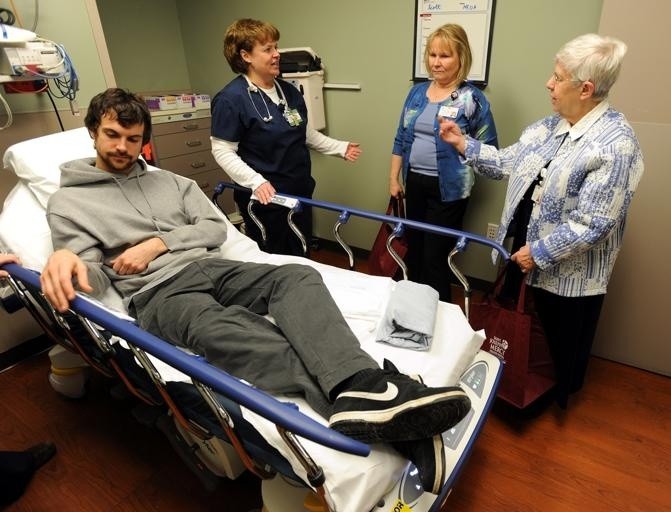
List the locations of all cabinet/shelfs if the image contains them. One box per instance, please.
[148,89,239,227]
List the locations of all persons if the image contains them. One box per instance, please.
[40,87,471,494]
[208,17,364,259]
[0,252,57,511]
[439,34,646,397]
[388,24,499,304]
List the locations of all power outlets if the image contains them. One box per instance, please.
[2,81,48,94]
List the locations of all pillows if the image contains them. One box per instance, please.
[0,127,151,213]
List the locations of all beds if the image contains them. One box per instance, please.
[0,126,512,512]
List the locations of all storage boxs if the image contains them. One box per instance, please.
[276,45,327,142]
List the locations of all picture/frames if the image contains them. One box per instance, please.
[409,0,498,89]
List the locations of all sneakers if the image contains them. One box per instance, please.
[327,359,473,445]
[391,433,447,496]
